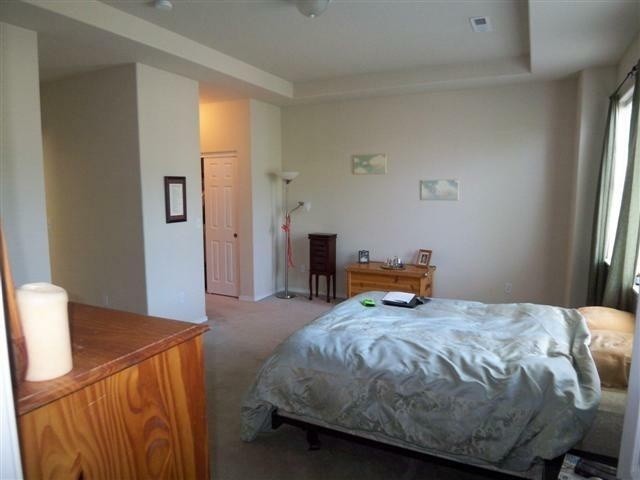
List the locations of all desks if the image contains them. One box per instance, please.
[15,302,212,480]
[344,261,436,299]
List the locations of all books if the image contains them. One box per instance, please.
[558,453,617,480]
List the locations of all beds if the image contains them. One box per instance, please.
[239,291,636,480]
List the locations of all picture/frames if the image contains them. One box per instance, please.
[416,249,432,268]
[359,250,369,264]
[164,176,187,223]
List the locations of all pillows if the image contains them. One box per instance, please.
[583,330,632,390]
[576,306,634,333]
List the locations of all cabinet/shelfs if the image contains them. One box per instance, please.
[308,233,337,303]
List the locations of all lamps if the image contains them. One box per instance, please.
[294,0,328,18]
[275,170,311,300]
